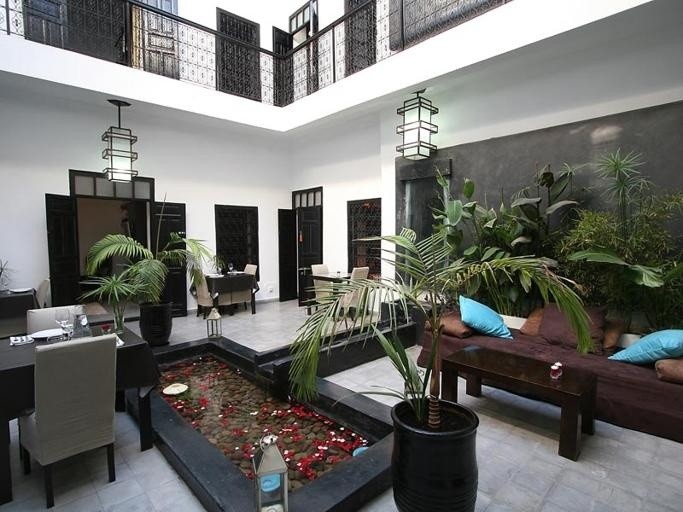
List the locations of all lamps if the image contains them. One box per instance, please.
[396,87,439,162]
[101,99,137,183]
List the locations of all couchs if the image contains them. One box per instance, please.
[416,313,682,434]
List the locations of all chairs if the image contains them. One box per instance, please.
[26,304,84,334]
[243,263,257,309]
[348,266,369,320]
[18,332,116,508]
[193,269,214,319]
[311,264,335,310]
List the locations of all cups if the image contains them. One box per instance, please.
[554,361,562,376]
[550,365,558,379]
[74,305,86,329]
[227,263,233,273]
[55,308,69,333]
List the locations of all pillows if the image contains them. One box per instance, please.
[458,295,513,340]
[538,304,603,356]
[607,329,682,365]
[425,309,472,339]
[519,307,545,336]
[602,319,624,354]
[655,358,682,384]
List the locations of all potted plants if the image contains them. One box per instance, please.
[75,268,151,334]
[85,231,212,347]
[287,227,592,512]
